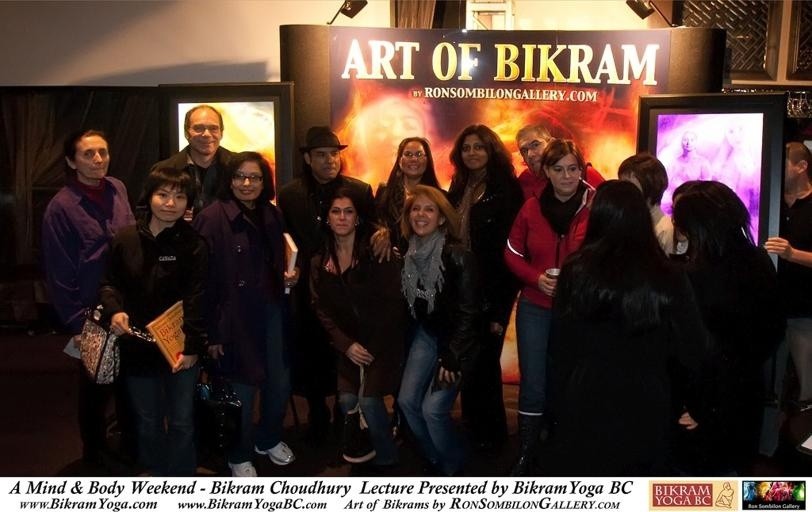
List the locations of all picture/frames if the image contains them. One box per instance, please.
[635,90,789,274]
[157,79,297,209]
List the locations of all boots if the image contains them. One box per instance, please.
[508,412,543,477]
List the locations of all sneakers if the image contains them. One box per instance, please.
[228,461,258,477]
[254,440,296,466]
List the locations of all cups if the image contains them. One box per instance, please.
[731,88,811,119]
[545,268,561,279]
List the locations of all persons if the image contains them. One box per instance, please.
[762,142,812,402]
[38,127,139,476]
[96,107,777,476]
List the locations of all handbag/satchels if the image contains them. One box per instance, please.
[343,402,377,463]
[80,318,121,384]
[194,378,243,410]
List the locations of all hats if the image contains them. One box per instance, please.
[299,126,348,156]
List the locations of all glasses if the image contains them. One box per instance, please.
[404,151,426,159]
[131,326,156,344]
[233,174,264,183]
